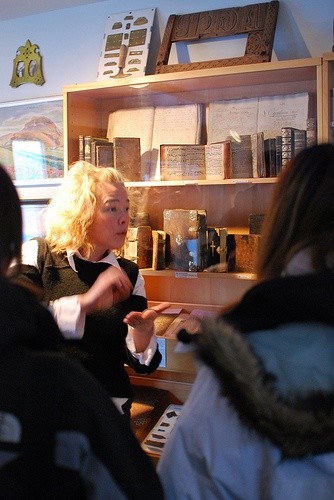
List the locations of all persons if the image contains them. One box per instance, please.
[0,163,73,500]
[10,161,162,427]
[156,142,334,500]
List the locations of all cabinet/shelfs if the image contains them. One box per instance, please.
[63,52,334,408]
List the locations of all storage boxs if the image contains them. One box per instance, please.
[151,314,208,376]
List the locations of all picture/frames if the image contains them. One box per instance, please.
[0,95,63,188]
[20,198,53,244]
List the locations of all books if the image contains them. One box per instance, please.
[123,209,265,274]
[154,312,205,375]
[140,404,183,457]
[78,91,317,180]
